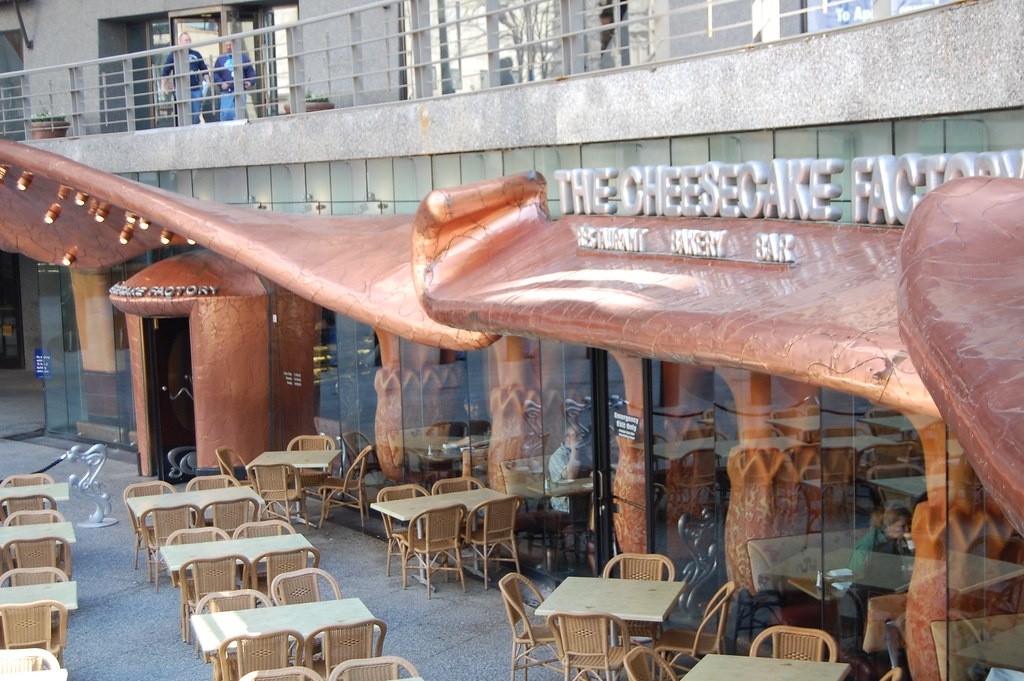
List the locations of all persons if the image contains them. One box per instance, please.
[848,504,916,661]
[548,423,595,527]
[160,32,211,125]
[449,392,484,470]
[213,41,256,122]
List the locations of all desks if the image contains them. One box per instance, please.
[126,485,267,527]
[681,653,852,681]
[0,581,78,614]
[247,451,339,530]
[157,534,317,573]
[191,598,385,653]
[0,483,71,504]
[788,572,868,654]
[372,487,505,594]
[1,521,76,548]
[534,577,686,681]
[410,442,465,465]
[531,477,591,556]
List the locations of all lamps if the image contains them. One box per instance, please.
[125,211,139,223]
[44,212,58,223]
[120,232,133,246]
[74,192,89,206]
[139,219,153,230]
[159,229,174,244]
[61,252,75,266]
[18,178,30,191]
[93,208,108,222]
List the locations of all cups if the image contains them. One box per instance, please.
[903,533,916,550]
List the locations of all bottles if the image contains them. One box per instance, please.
[428,443,432,456]
[816,570,822,588]
[543,475,553,489]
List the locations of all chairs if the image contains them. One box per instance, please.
[0,474,53,484]
[0,495,56,518]
[122,435,902,681]
[0,567,69,587]
[1,537,72,578]
[0,648,59,672]
[0,509,65,526]
[0,601,67,670]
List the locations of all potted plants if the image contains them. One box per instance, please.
[303,93,333,112]
[31,112,70,138]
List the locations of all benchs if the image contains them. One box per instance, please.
[461,449,487,484]
[930,613,1024,681]
[745,527,872,631]
[862,593,911,681]
[382,425,454,487]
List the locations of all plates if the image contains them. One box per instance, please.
[558,479,574,484]
[583,483,594,488]
